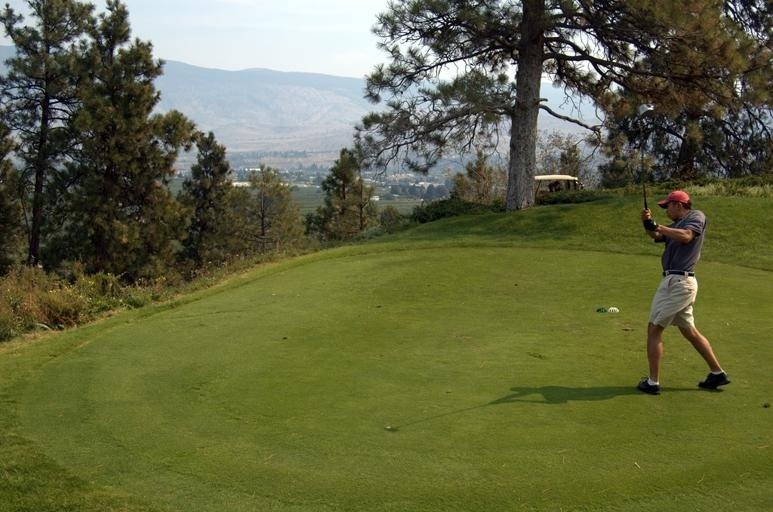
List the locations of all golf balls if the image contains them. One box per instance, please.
[608,307,619,312]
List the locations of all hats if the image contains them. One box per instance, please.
[657,191,690,209]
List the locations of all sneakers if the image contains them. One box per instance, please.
[698,372,730,389]
[637,377,660,392]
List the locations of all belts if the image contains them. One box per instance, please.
[662,271,694,276]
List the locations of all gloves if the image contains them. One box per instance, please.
[643,219,658,231]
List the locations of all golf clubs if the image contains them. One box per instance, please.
[638,103,654,210]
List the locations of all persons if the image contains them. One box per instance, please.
[638,191,732,396]
[547,183,555,193]
[554,182,561,192]
